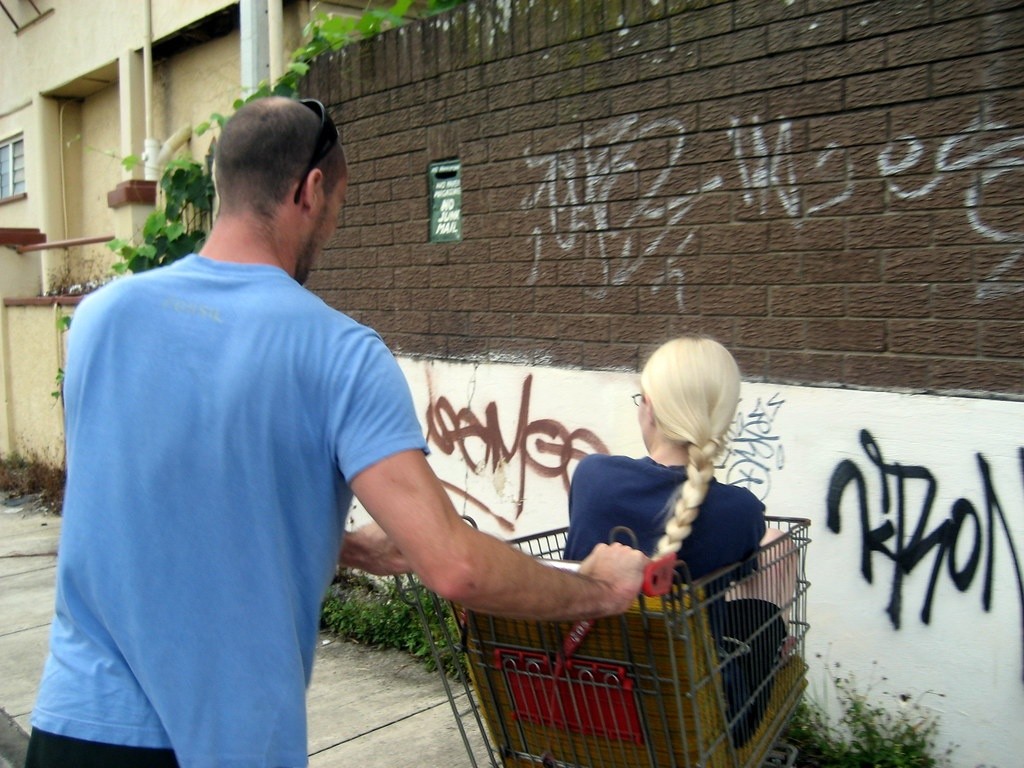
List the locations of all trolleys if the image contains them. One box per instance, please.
[428,512,809,767]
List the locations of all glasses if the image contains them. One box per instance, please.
[633,394,645,407]
[294,98,338,205]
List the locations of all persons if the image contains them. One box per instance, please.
[20,93,654,768]
[561,335,789,750]
